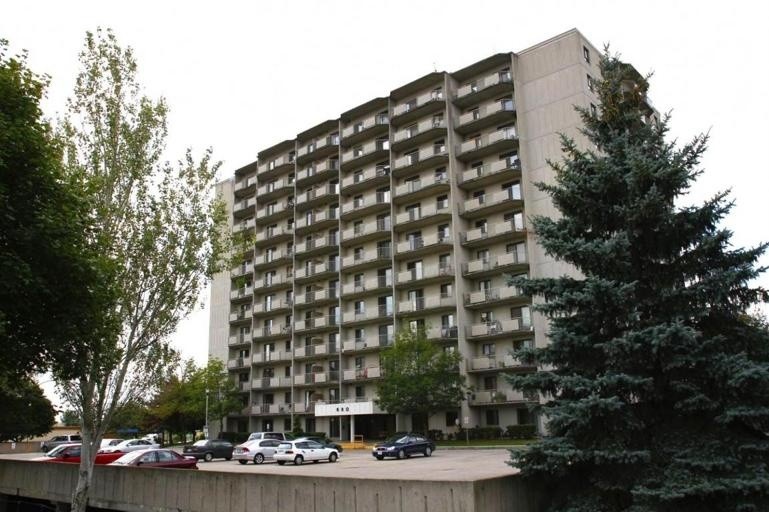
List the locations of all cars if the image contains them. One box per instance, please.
[232,430,343,463]
[373,431,434,459]
[181,438,235,462]
[36,432,198,469]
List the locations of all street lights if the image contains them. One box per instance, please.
[204,388,210,438]
